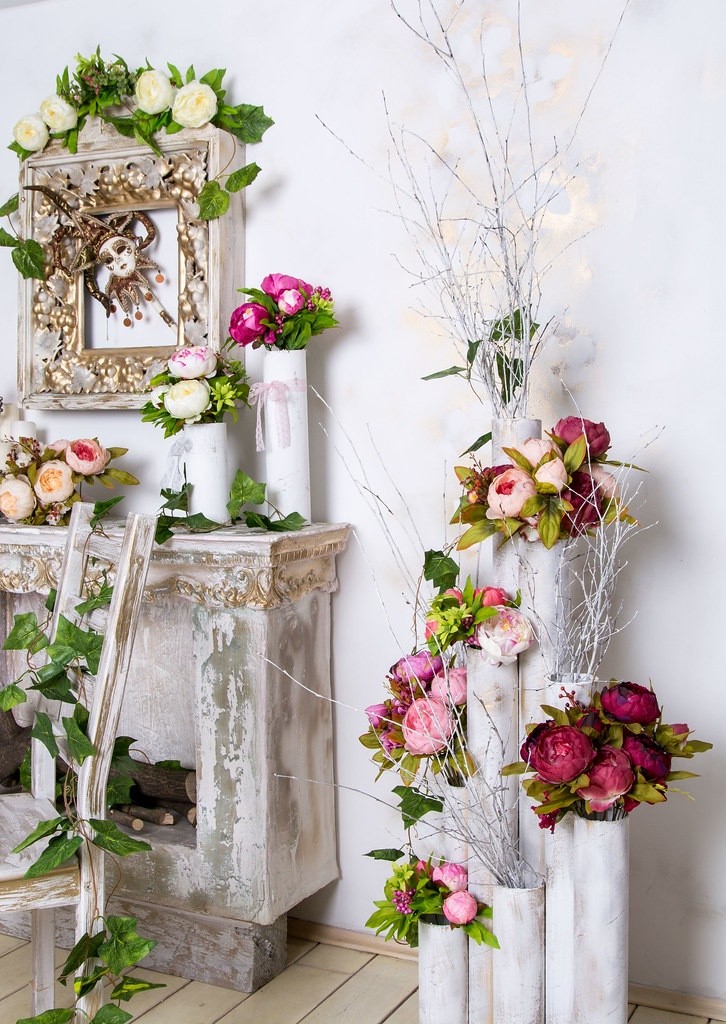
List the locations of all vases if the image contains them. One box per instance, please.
[183,422,231,526]
[418,427,630,1024]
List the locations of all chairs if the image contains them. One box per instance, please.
[0,500,158,1024]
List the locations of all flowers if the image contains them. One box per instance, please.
[0,44,274,280]
[139,344,252,440]
[0,435,141,526]
[261,350,311,526]
[220,274,341,352]
[365,418,714,950]
